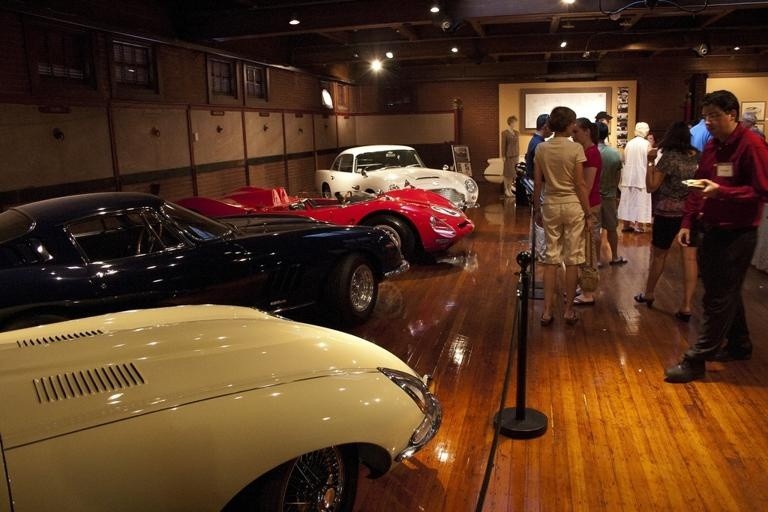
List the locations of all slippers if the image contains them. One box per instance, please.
[541,255,627,326]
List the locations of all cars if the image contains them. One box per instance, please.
[309,145,482,212]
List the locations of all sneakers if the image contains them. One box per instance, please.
[665,359,704,381]
[714,341,752,361]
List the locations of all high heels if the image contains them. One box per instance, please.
[674,311,691,322]
[633,294,653,307]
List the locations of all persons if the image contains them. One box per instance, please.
[502,116,519,197]
[525,107,628,326]
[617,112,766,232]
[664,89,768,383]
[635,122,701,322]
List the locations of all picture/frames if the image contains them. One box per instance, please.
[754,123,766,134]
[742,101,766,120]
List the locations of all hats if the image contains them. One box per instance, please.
[595,112,612,120]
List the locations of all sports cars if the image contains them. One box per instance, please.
[0,193,415,317]
[0,303,444,510]
[170,186,476,261]
